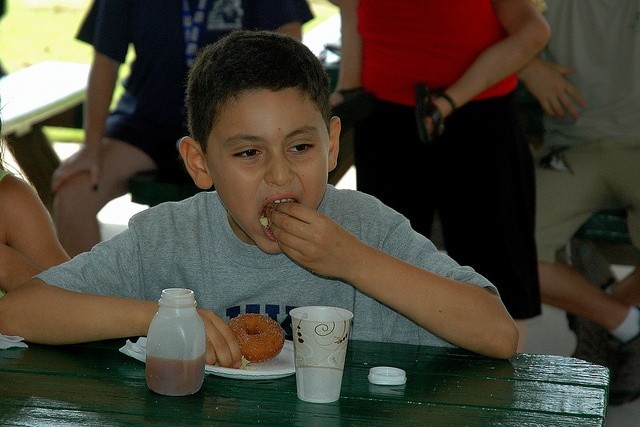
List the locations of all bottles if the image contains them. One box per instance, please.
[145,288,206,396]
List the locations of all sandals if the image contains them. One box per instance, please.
[416,84,456,146]
[329,87,381,127]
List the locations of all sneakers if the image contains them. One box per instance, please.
[593,333,640,405]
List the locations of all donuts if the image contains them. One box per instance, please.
[226,311,287,363]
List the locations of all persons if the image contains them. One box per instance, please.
[1,101,73,289]
[354,0,541,354]
[518,0,640,402]
[49,2,316,259]
[324,0,552,143]
[1,26,519,361]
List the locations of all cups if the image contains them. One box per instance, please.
[290,307,354,403]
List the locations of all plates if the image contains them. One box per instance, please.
[206,340,296,379]
